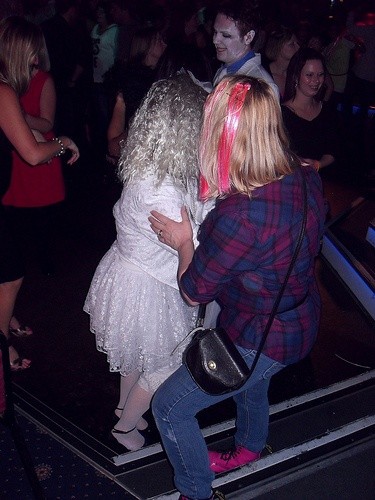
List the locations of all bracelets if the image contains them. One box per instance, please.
[313,160,319,172]
[52,138,64,157]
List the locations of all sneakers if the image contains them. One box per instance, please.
[203,444,262,473]
[178,487,226,500]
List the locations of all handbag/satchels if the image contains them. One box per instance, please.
[182,328,252,396]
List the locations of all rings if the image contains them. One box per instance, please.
[159,230,162,236]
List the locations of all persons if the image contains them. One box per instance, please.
[82,77,219,451]
[149,74,328,500]
[0,0,374,372]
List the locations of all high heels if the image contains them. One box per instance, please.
[8,323,32,338]
[109,407,153,436]
[6,343,32,372]
[109,426,151,455]
